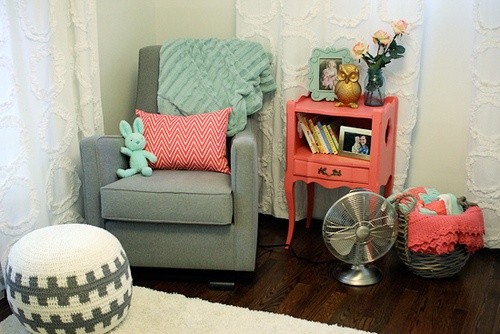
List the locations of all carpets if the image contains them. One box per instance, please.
[0,284,382,334]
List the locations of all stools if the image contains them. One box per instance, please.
[0,222,135,334]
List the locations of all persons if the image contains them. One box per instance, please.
[351,135,369,155]
[322,59,337,90]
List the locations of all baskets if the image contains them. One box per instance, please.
[393,192,484,278]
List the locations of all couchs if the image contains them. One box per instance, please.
[76,42,262,292]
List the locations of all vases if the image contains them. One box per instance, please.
[362,67,389,109]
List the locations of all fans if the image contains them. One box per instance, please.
[321,187,399,287]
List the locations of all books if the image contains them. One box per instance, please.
[296,112,342,155]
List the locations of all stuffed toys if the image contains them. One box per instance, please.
[116,117,157,179]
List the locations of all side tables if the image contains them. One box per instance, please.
[280,91,402,253]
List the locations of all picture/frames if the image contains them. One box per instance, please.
[303,44,344,103]
[336,126,373,160]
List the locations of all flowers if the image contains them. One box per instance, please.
[352,18,413,104]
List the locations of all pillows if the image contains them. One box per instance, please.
[134,107,232,176]
